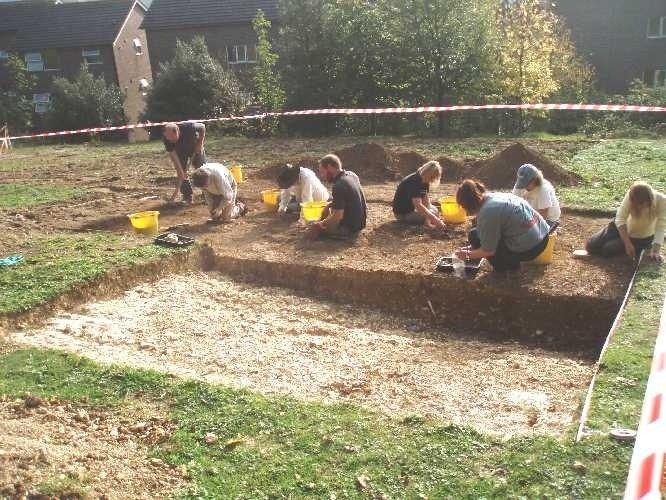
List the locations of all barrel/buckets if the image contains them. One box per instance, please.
[438,193,466,224]
[127,211,160,236]
[299,201,330,221]
[229,164,243,184]
[260,188,282,205]
[524,231,555,266]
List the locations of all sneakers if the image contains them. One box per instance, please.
[490,270,518,279]
[235,200,248,216]
[181,190,207,206]
[327,232,348,240]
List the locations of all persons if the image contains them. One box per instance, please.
[161,121,207,205]
[454,180,551,277]
[275,162,331,231]
[306,153,369,243]
[586,180,666,262]
[510,163,562,233]
[191,162,248,223]
[391,161,448,234]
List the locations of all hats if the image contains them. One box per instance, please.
[515,163,538,188]
[277,164,299,189]
